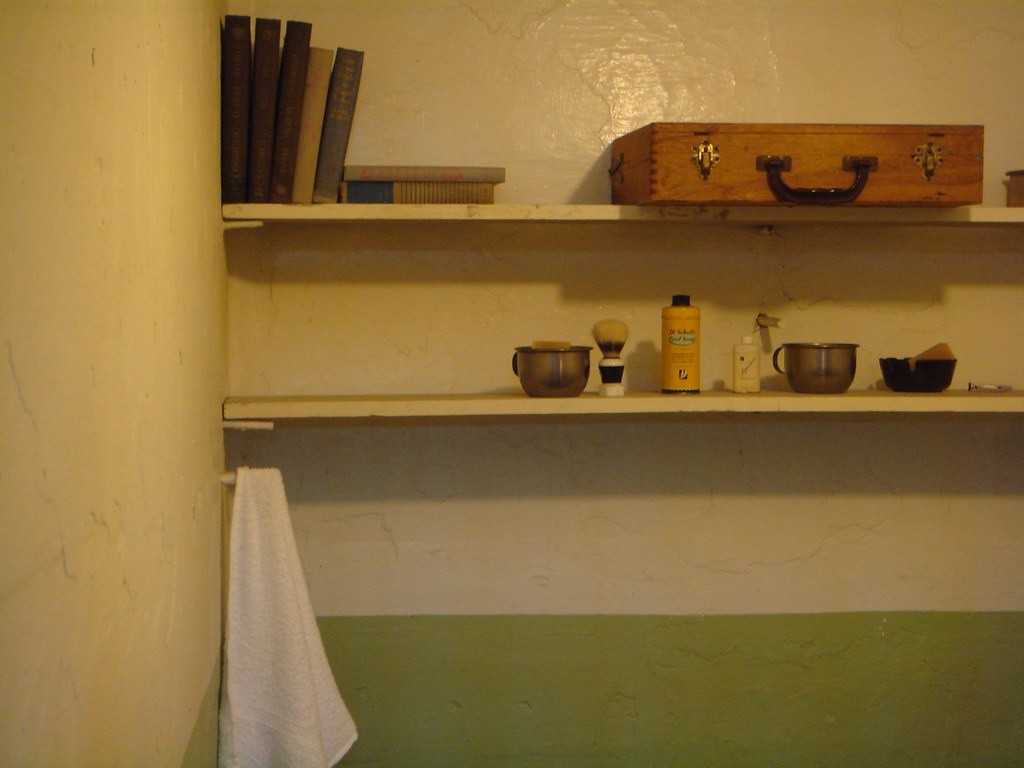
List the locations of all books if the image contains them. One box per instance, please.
[222,12,504,204]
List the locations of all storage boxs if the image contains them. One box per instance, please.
[609,123,985,206]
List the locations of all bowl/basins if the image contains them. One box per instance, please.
[772,343,860,394]
[511,346,593,398]
[880,357,957,393]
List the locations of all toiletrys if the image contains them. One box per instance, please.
[732,335,760,393]
[661,294,701,395]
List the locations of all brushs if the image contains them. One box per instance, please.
[591,318,633,396]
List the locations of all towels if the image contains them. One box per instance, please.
[221,466,359,768]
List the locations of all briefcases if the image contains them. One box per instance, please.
[610,121,985,206]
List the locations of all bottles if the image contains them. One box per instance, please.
[732,335,761,394]
[662,294,701,393]
